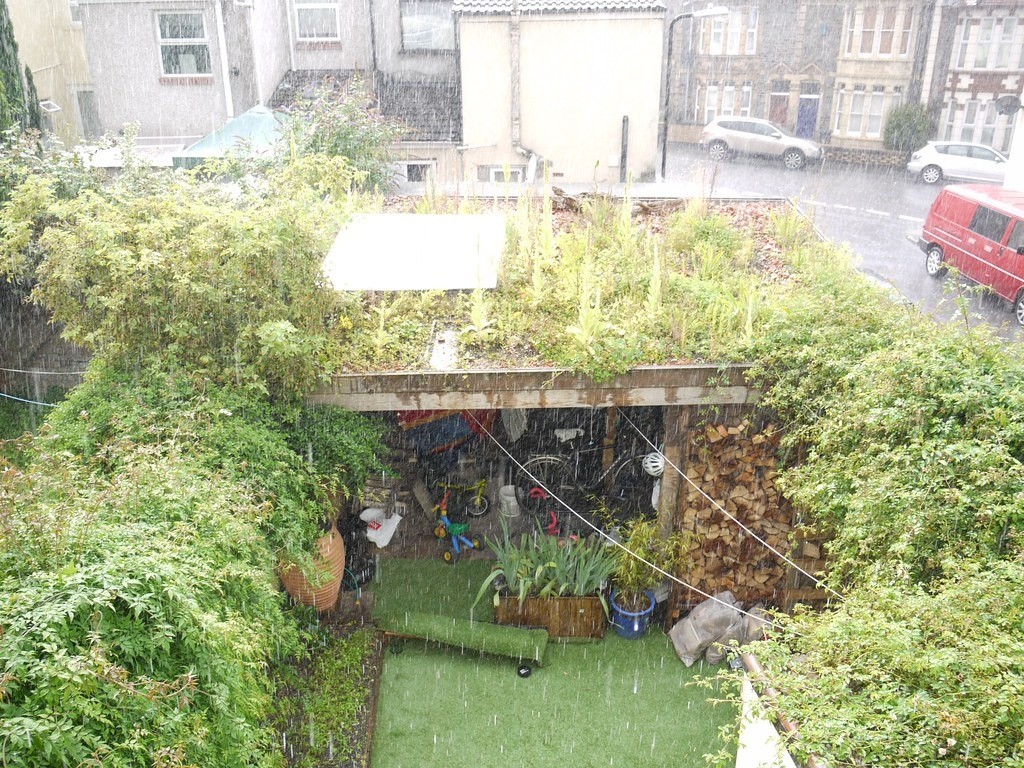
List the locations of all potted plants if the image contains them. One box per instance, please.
[269,468,346,613]
[472,494,624,645]
[584,492,703,640]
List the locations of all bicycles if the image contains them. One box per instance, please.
[516,423,656,520]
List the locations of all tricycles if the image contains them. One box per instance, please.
[436,466,491,518]
[431,482,485,565]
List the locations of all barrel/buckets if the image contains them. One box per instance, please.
[500,485,523,517]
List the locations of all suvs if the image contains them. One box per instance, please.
[700,116,823,170]
[907,140,1007,185]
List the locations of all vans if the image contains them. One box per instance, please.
[919,181,1024,325]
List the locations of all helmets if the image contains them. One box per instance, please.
[642,452,665,476]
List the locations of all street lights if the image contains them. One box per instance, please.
[662,6,731,180]
